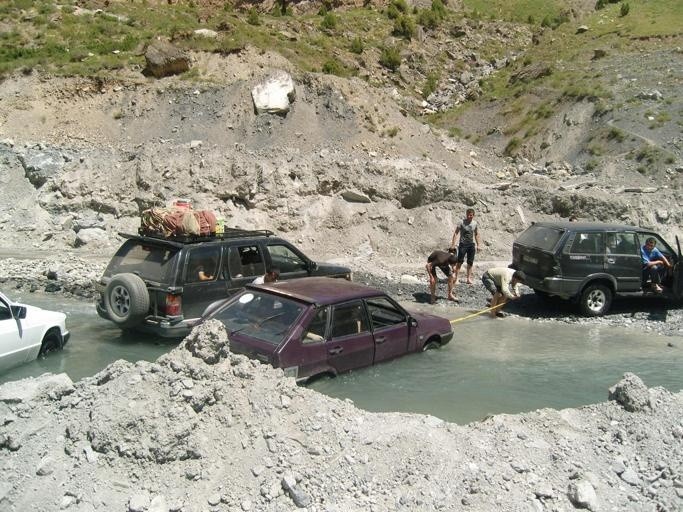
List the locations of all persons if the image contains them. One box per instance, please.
[640,237,672,292]
[600,234,615,254]
[482,267,525,317]
[251,271,278,286]
[451,209,481,284]
[425,250,460,303]
[195,264,213,280]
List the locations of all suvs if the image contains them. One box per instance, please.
[507,222,683,316]
[94,225,351,338]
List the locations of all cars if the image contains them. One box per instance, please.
[0,347,68,385]
[188,276,454,388]
[0,291,69,375]
